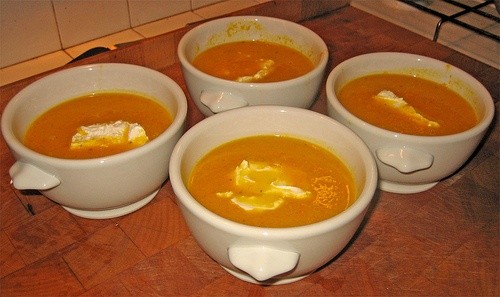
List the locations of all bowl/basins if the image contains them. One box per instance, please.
[175,15,329,118]
[324,51,495,195]
[168,103,381,285]
[0,62,188,220]
